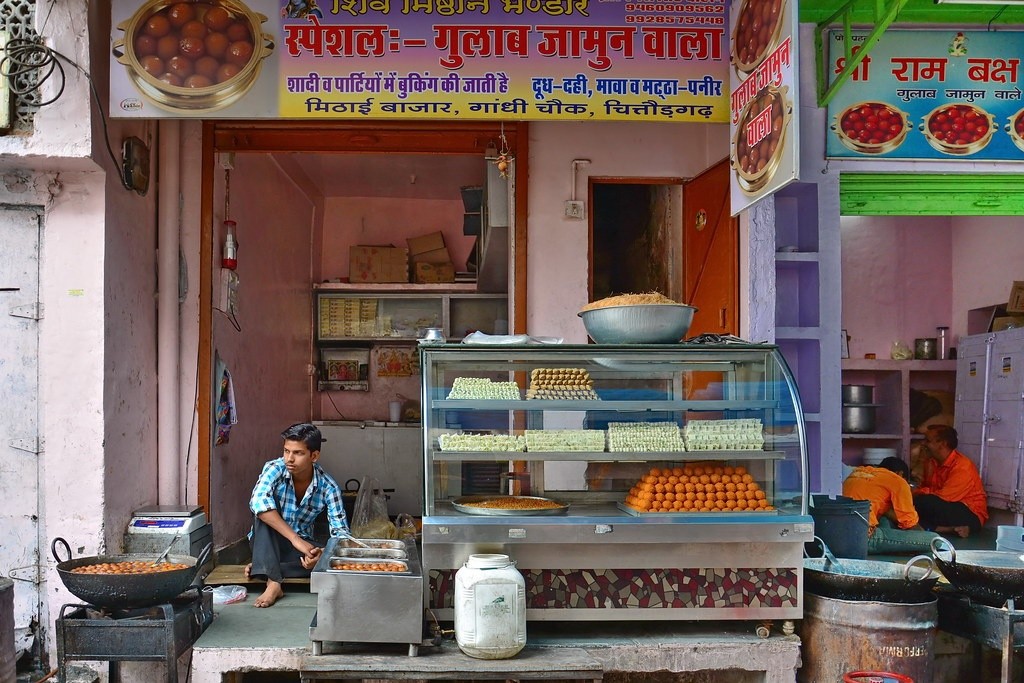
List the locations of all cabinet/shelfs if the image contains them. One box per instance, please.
[775,183,823,502]
[314,290,509,391]
[417,343,815,637]
[840,357,956,486]
[312,424,510,517]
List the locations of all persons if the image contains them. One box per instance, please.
[841,457,940,550]
[248,423,350,608]
[906,424,990,539]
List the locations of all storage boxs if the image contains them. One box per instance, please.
[992,316,1024,333]
[320,298,392,338]
[1004,280,1024,314]
[347,230,456,283]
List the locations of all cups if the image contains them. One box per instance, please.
[937,326,950,360]
[388,401,402,422]
[914,338,937,360]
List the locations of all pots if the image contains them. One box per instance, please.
[803,535,941,604]
[930,538,1024,609]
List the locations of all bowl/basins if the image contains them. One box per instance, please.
[111,1,276,111]
[1004,107,1024,153]
[728,0,786,81]
[424,327,446,344]
[730,83,794,198]
[831,100,913,155]
[51,537,215,609]
[578,306,699,346]
[918,102,1000,156]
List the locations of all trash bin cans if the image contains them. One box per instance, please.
[782,495,876,559]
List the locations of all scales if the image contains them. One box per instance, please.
[128,505,206,534]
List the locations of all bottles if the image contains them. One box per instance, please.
[950,347,957,359]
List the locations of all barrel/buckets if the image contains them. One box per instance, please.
[804,494,872,558]
[863,447,897,465]
[841,384,884,434]
[454,553,527,660]
[796,591,939,683]
